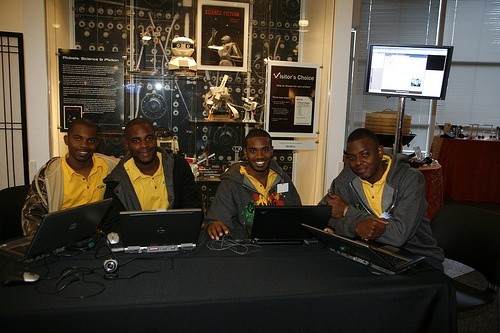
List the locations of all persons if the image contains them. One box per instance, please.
[325,128,442,261]
[21,118,120,235]
[206,129,302,241]
[103,118,205,234]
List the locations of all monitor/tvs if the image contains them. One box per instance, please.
[362,43,454,100]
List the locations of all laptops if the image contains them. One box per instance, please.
[0,197,112,259]
[246,205,333,244]
[120,209,202,253]
[300,221,427,274]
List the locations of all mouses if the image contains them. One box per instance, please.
[218,232,232,240]
[105,232,119,245]
[6,270,39,285]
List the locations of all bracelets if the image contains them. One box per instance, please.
[343,206,349,217]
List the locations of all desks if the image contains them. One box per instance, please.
[0,229,460,333]
[418,163,444,221]
[431,136,500,203]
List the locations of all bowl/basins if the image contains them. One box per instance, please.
[407,159,425,169]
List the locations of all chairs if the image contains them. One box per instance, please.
[430,205,500,333]
[0,186,31,244]
